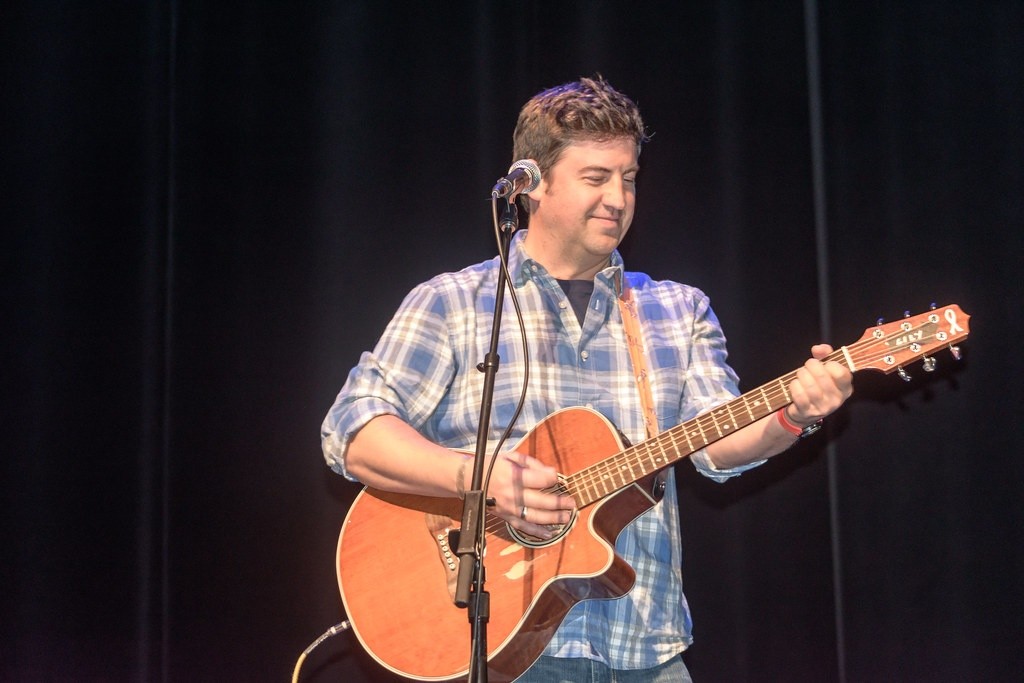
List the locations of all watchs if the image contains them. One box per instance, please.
[778,408,823,438]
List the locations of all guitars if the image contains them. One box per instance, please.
[335,303,971,682]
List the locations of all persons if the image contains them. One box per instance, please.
[321,80,852,683]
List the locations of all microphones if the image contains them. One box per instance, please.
[491,159,541,198]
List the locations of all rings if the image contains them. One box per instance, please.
[521,506,527,518]
